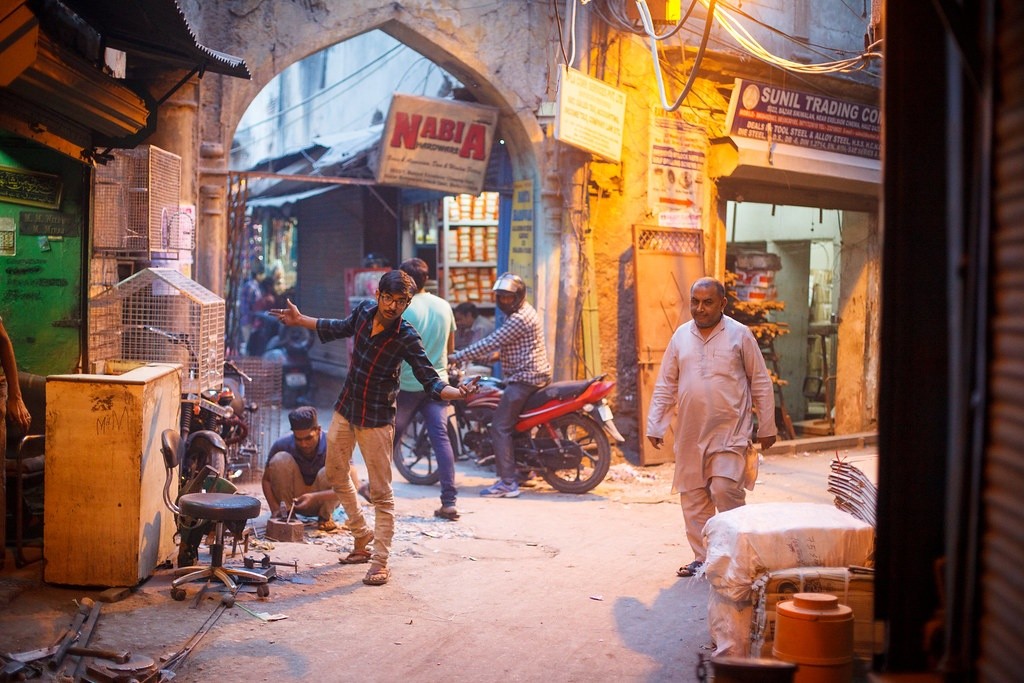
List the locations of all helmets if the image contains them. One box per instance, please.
[492,273,526,314]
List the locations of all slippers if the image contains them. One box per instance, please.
[363,568,391,584]
[317,516,338,531]
[434,507,460,519]
[339,548,374,564]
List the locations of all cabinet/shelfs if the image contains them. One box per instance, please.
[414,198,501,309]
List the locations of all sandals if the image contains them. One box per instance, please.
[676,561,706,577]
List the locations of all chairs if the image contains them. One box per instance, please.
[161,427,269,607]
[5,371,48,565]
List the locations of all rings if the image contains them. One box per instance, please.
[280,309,282,314]
[26,417,30,419]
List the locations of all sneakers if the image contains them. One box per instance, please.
[480,481,520,497]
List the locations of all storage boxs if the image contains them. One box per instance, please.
[733,262,780,302]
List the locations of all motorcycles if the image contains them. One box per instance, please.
[168,331,260,492]
[394,361,626,495]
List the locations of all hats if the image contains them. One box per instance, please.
[289,406,319,430]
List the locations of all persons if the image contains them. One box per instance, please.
[448,271,552,499]
[0,317,31,434]
[240,264,285,343]
[455,302,495,350]
[646,276,778,577]
[262,405,359,531]
[268,270,480,585]
[357,259,460,519]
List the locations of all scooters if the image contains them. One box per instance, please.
[264,324,317,410]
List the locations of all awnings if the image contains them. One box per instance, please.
[63,0,252,156]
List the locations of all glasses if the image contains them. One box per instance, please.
[379,291,411,310]
